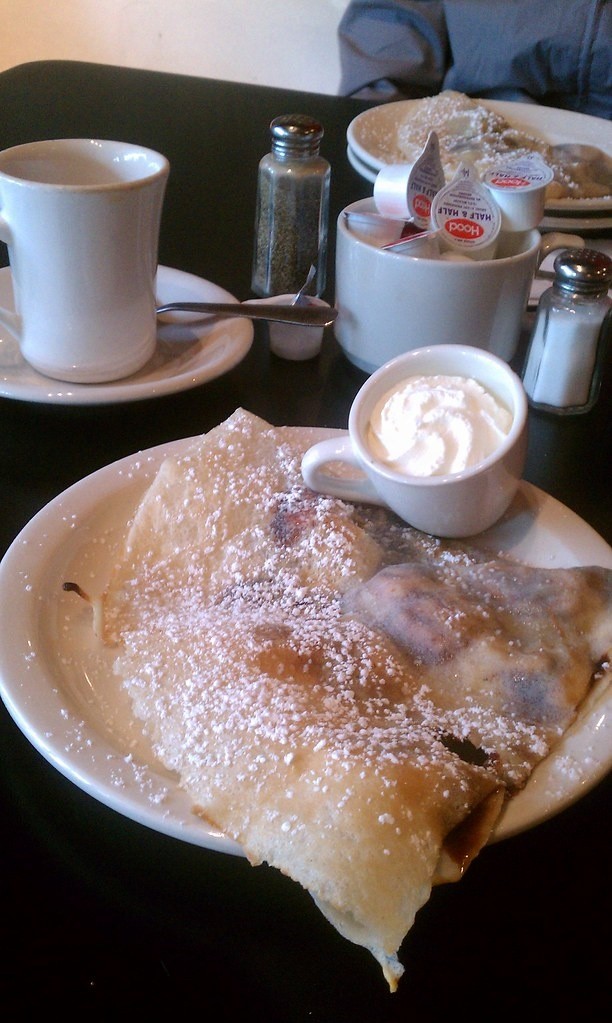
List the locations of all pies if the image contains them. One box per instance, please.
[400,88,612,199]
[106,406,612,993]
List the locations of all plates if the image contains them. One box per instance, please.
[346,98,612,233]
[0,426,612,858]
[0,265,255,406]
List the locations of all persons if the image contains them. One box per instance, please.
[338,0,611,126]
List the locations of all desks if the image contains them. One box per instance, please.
[0,60,612,1023]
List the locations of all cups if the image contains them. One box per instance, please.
[331,196,585,375]
[302,344,528,538]
[1,139,170,385]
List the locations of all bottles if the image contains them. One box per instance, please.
[521,249,612,416]
[251,114,331,298]
